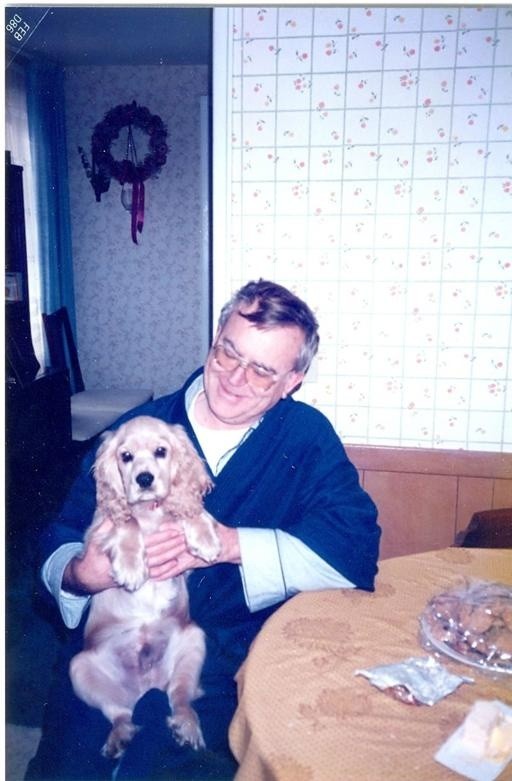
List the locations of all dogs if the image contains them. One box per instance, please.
[67,413,222,761]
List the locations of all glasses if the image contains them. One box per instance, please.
[214,323,300,390]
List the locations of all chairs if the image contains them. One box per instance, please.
[42,305,154,466]
[450,508,512,549]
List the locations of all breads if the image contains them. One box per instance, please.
[463,701,512,761]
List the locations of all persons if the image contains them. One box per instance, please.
[20,277,385,781]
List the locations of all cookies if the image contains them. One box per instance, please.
[424,580,512,670]
